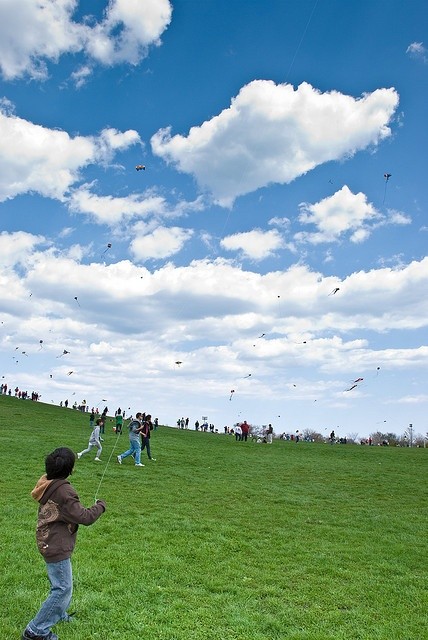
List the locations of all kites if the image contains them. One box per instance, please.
[66,371,74,376]
[22,352,28,357]
[57,349,70,358]
[259,334,265,338]
[38,340,43,351]
[103,244,111,254]
[346,385,357,391]
[355,378,364,383]
[74,297,79,306]
[244,374,251,378]
[175,361,182,365]
[383,173,391,203]
[136,165,146,171]
[230,390,234,401]
[328,288,339,295]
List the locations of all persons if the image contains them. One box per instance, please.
[77,420,104,461]
[32,391,39,401]
[195,421,214,433]
[257,437,267,443]
[91,407,99,414]
[117,413,146,466]
[150,417,158,430]
[235,423,242,441]
[268,424,273,444]
[295,430,300,443]
[99,413,106,433]
[23,447,105,639]
[329,431,335,444]
[90,413,94,426]
[360,437,372,445]
[240,421,249,441]
[15,387,27,399]
[0,384,11,396]
[131,415,156,461]
[224,426,234,433]
[116,411,123,435]
[177,418,189,429]
[72,400,88,412]
[60,400,68,408]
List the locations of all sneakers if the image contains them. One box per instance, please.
[117,456,122,464]
[77,453,82,459]
[95,457,102,461]
[61,612,75,622]
[131,455,134,459]
[135,463,145,466]
[22,630,58,640]
[148,458,156,461]
